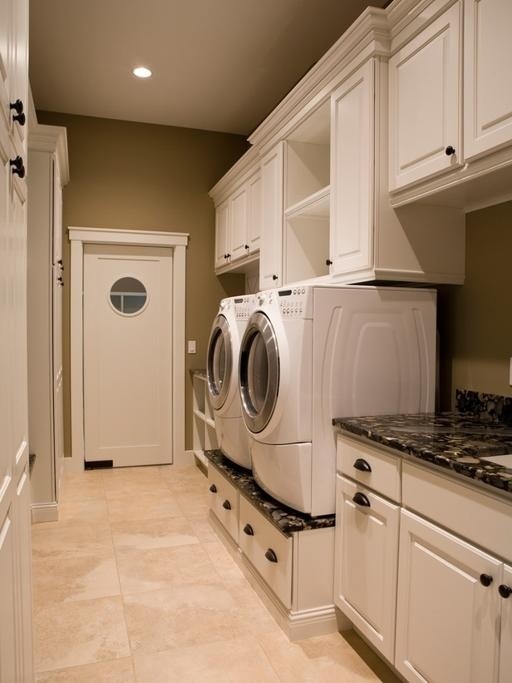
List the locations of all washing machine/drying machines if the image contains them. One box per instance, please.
[205,293,255,469]
[236,284,439,519]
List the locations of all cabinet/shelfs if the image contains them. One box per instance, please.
[245,147,258,275]
[1,0,34,682]
[331,419,401,668]
[246,8,465,289]
[206,450,336,640]
[386,0,512,214]
[27,126,70,523]
[396,441,512,683]
[208,150,248,277]
[192,370,215,479]
